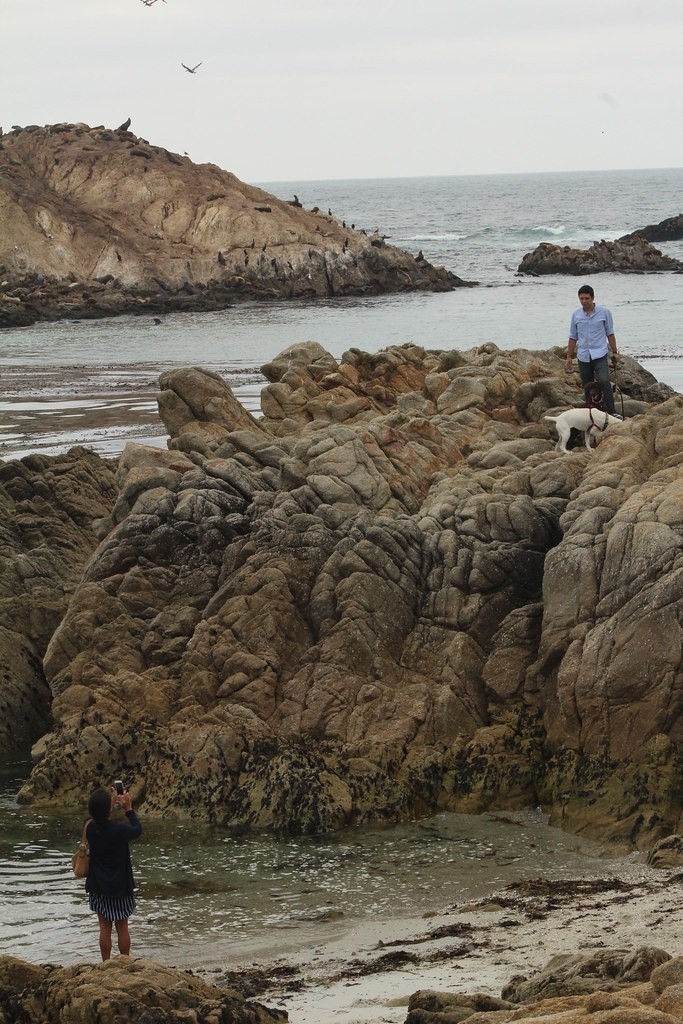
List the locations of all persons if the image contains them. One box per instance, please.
[566,285,620,413]
[84,786,141,961]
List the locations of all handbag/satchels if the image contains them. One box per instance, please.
[72,819,93,879]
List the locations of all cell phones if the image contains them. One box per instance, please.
[115,780,125,795]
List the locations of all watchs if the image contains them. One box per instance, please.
[613,351,619,354]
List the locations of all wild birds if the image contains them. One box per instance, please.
[243,238,277,268]
[218,251,225,266]
[293,194,332,216]
[140,0,168,7]
[181,62,202,73]
[308,248,312,260]
[343,238,348,255]
[287,261,294,270]
[115,251,122,262]
[342,220,386,241]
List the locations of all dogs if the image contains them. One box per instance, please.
[543,407,623,456]
